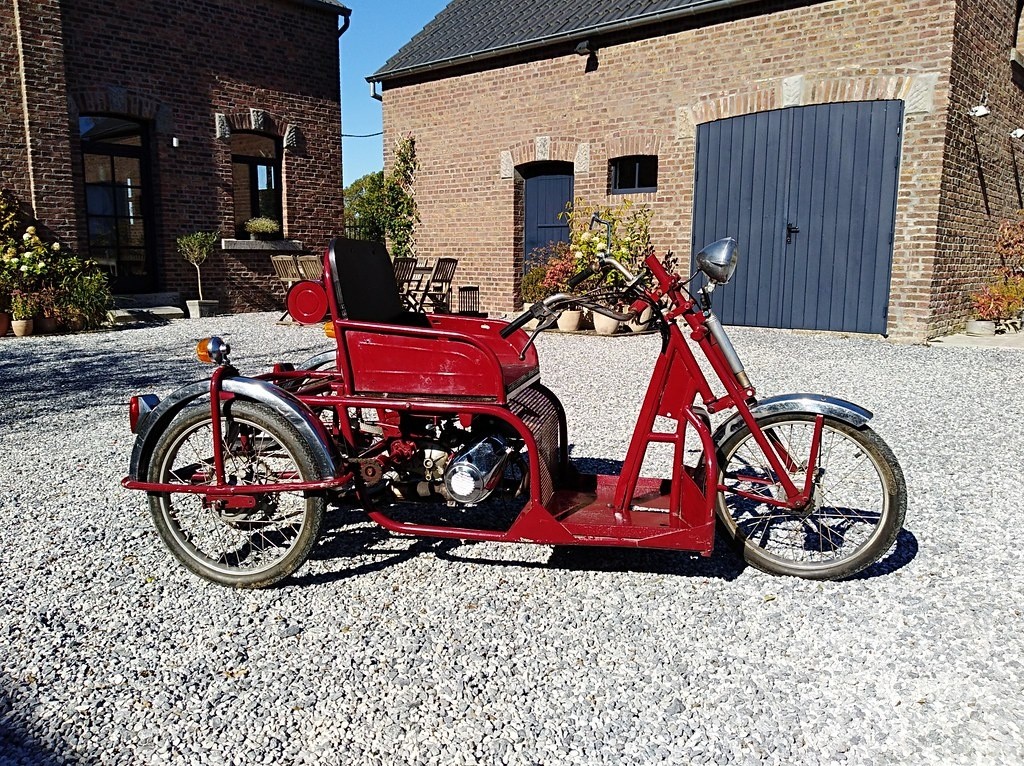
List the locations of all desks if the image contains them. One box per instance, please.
[414,266,433,275]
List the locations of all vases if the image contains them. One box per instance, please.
[554,311,581,333]
[11,320,33,338]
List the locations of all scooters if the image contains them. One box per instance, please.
[121,212,907,589]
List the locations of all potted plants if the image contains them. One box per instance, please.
[967,209,1024,337]
[520,196,653,335]
[176,231,219,318]
[0,186,114,337]
[244,218,280,241]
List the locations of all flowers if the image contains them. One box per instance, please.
[5,289,40,319]
[543,260,576,310]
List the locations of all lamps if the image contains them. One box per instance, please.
[967,106,991,118]
[573,40,595,56]
[168,133,179,148]
[1007,128,1024,139]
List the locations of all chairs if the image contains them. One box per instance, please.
[324,237,566,511]
[392,256,458,312]
[270,254,324,326]
